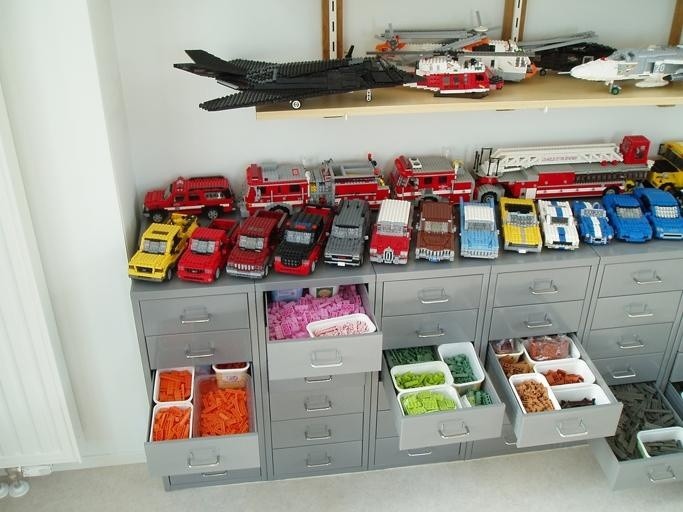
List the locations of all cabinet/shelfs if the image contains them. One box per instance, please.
[128,235,683,496]
